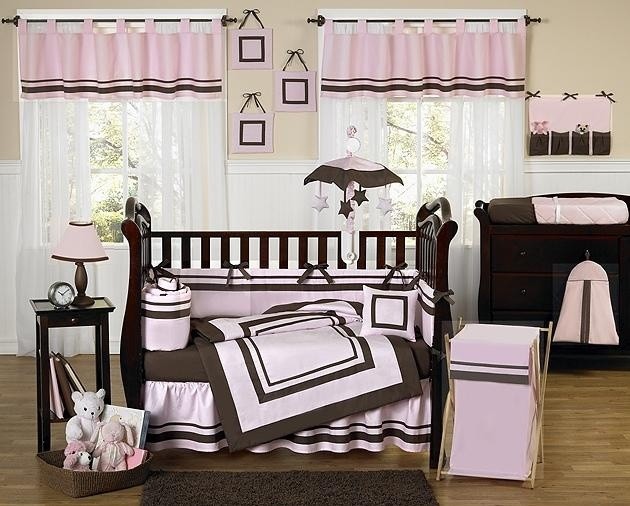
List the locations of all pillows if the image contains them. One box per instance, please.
[360,283,422,345]
[531,194,629,226]
[486,196,536,227]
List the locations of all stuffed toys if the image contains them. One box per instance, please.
[90,415,134,472]
[65,389,109,454]
[531,120,548,136]
[576,123,589,135]
[63,440,92,472]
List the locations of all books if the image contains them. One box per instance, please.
[48,350,86,420]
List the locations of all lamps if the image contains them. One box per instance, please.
[49,218,110,308]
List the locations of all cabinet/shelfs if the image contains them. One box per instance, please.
[472,190,630,372]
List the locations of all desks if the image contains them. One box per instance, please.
[27,295,116,457]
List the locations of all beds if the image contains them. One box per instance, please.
[120,191,461,473]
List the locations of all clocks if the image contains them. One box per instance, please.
[47,281,77,309]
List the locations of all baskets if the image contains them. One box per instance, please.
[34,446,155,499]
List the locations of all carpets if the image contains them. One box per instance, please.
[136,470,439,506]
